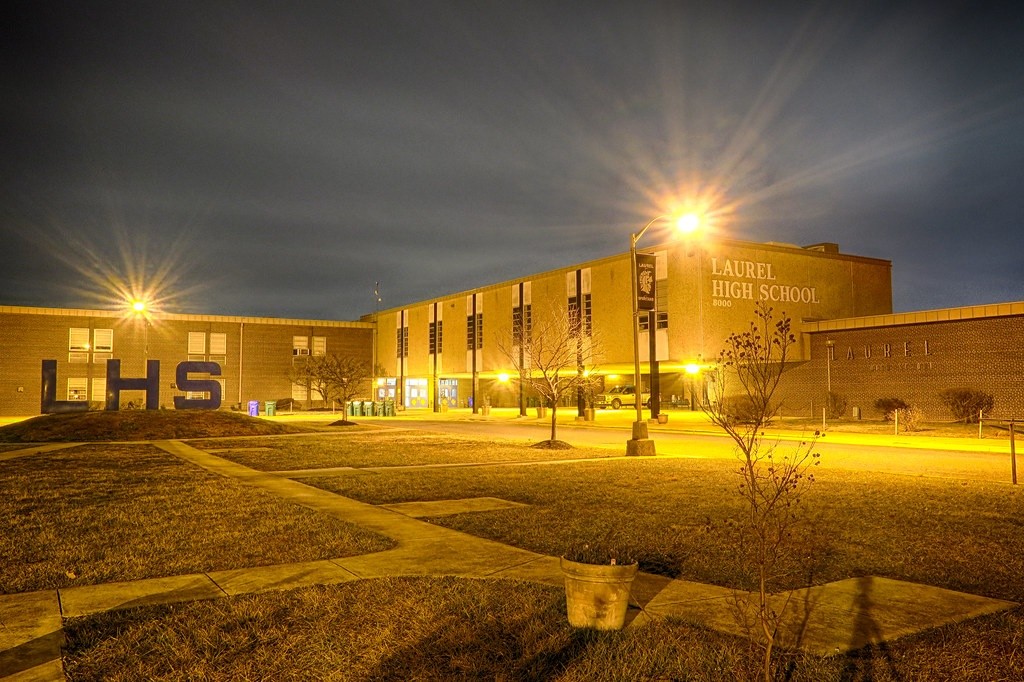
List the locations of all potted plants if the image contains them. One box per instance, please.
[657,412,670,424]
[581,385,596,421]
[481,393,491,416]
[536,387,548,418]
[440,396,448,412]
[558,532,639,628]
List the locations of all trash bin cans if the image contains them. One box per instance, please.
[249,400,259,416]
[535,395,542,407]
[385,401,394,416]
[468,396,474,407]
[374,401,385,416]
[346,401,353,416]
[526,395,535,407]
[353,400,364,416]
[364,401,374,416]
[265,400,276,416]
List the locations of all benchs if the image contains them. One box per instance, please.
[672,399,690,409]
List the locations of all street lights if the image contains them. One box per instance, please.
[632,213,696,439]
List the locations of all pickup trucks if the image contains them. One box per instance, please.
[594,384,663,411]
